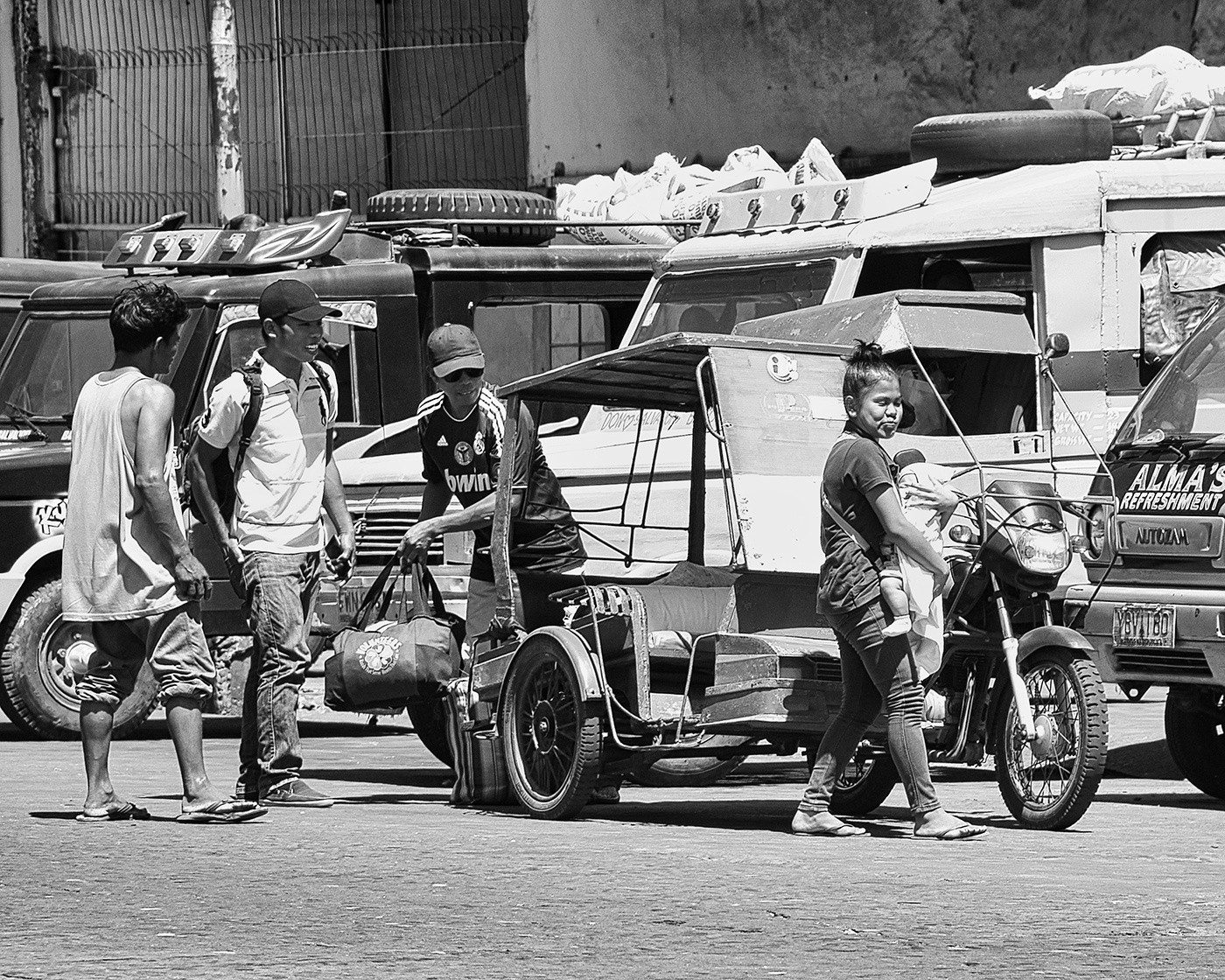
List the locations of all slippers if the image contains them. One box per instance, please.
[911,811,990,841]
[175,794,268,824]
[792,820,871,838]
[77,801,152,821]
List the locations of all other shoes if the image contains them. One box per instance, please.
[233,782,264,802]
[258,774,334,808]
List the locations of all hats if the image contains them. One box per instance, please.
[258,279,342,321]
[424,321,483,378]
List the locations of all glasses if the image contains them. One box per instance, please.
[442,368,484,386]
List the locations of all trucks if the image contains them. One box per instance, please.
[0,186,703,742]
[309,101,1225,788]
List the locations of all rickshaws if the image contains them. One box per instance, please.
[472,291,1120,833]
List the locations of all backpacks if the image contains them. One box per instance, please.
[181,358,333,526]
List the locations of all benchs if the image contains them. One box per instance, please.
[602,587,731,673]
[716,575,841,657]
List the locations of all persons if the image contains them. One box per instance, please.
[61,280,269,823]
[395,322,588,660]
[879,449,955,637]
[185,278,357,807]
[922,258,1037,436]
[791,339,990,841]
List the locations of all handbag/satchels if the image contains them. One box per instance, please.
[323,550,464,715]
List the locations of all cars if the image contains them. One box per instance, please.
[0,257,169,353]
[1062,305,1224,800]
